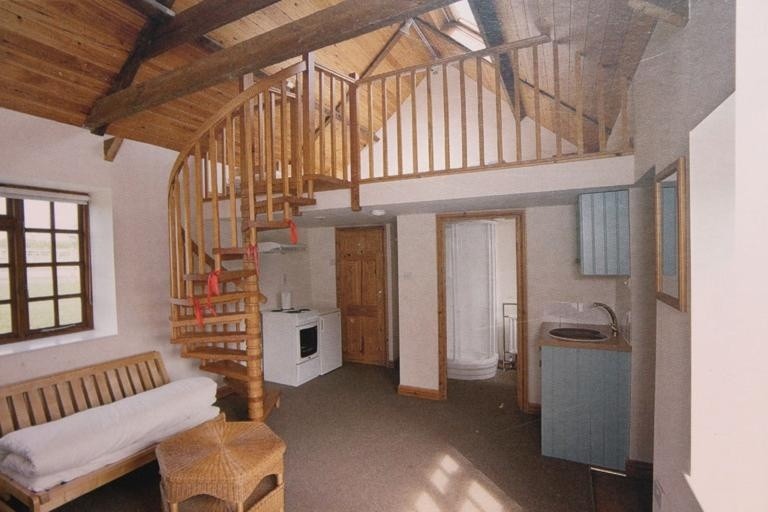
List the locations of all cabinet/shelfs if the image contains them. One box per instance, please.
[575,190,630,276]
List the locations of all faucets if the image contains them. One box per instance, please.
[593,302,619,336]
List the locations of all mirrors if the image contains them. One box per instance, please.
[653,156,685,311]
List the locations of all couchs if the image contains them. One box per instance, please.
[0,348,226,512]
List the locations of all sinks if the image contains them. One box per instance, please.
[549,328,608,342]
